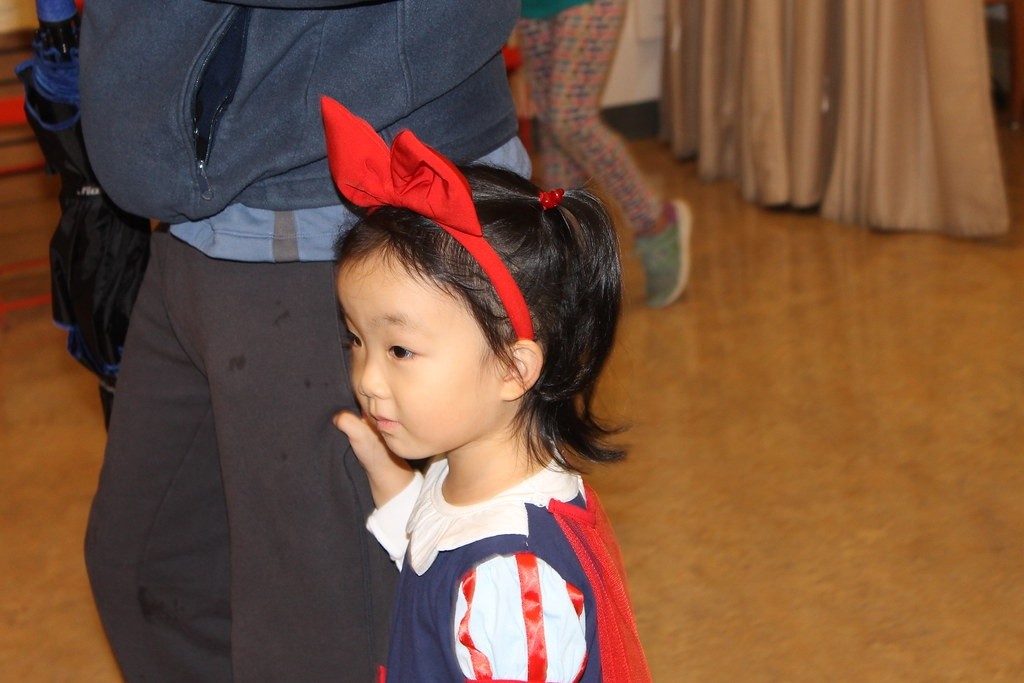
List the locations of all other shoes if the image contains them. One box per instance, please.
[634,197,691,310]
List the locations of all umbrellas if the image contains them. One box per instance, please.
[13,0,151,432]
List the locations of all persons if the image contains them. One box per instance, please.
[77,0,692,682]
